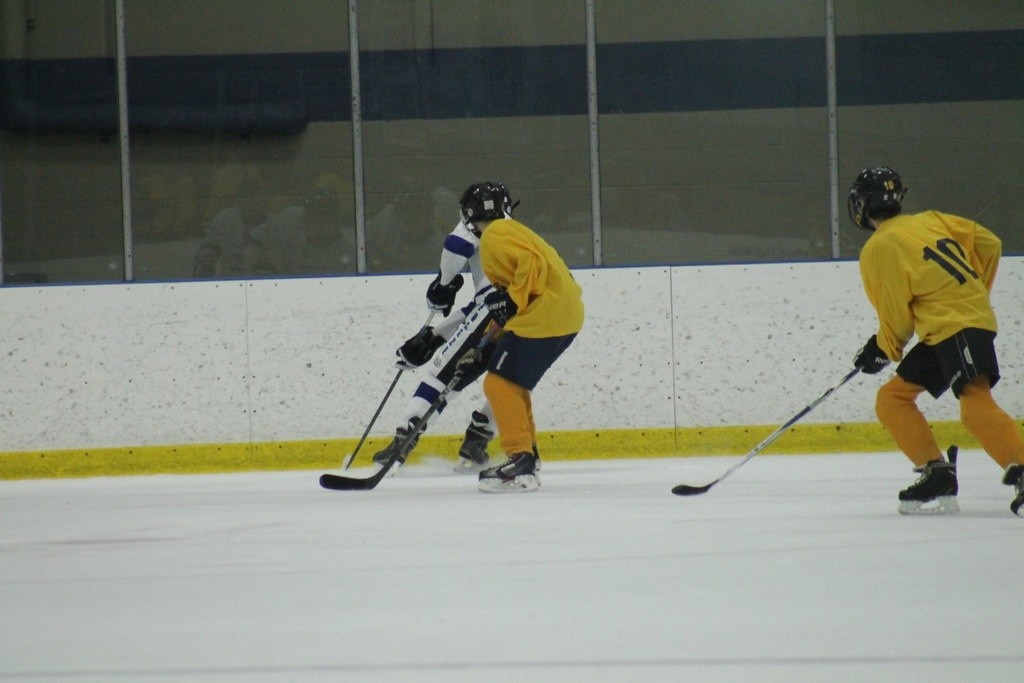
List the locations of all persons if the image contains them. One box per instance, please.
[847,165,1024,517]
[373,179,585,491]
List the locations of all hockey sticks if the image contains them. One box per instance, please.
[670,365,864,498]
[318,328,486,492]
[338,312,435,475]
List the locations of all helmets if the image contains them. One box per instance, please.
[495,182,520,216]
[459,181,505,230]
[847,166,908,231]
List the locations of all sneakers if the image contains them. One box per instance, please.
[1001,463,1024,517]
[897,445,960,515]
[479,444,542,494]
[453,410,494,475]
[372,416,427,479]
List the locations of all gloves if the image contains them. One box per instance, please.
[484,291,518,328]
[426,268,464,318]
[850,334,891,374]
[394,326,447,371]
[452,341,497,392]
